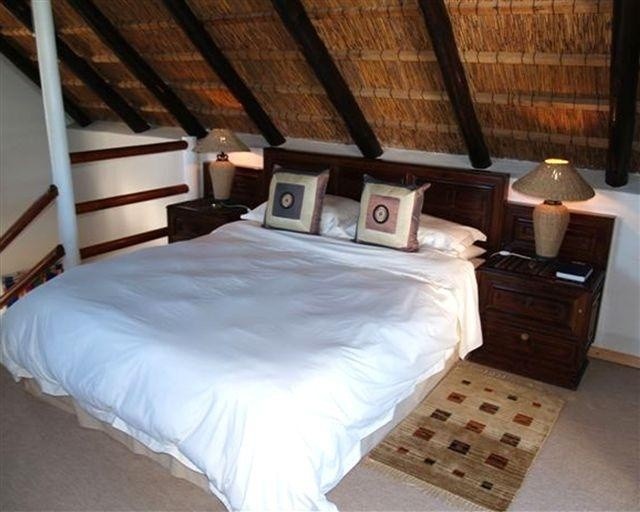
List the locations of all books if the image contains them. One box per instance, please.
[554,262,593,284]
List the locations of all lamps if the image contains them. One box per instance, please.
[511,158,596,260]
[191,128,251,203]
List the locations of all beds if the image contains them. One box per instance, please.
[0,144,510,512]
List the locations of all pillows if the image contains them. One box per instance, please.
[239,163,487,256]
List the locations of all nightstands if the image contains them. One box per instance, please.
[166,162,264,244]
[468,200,617,392]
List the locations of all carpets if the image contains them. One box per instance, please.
[358,357,576,512]
